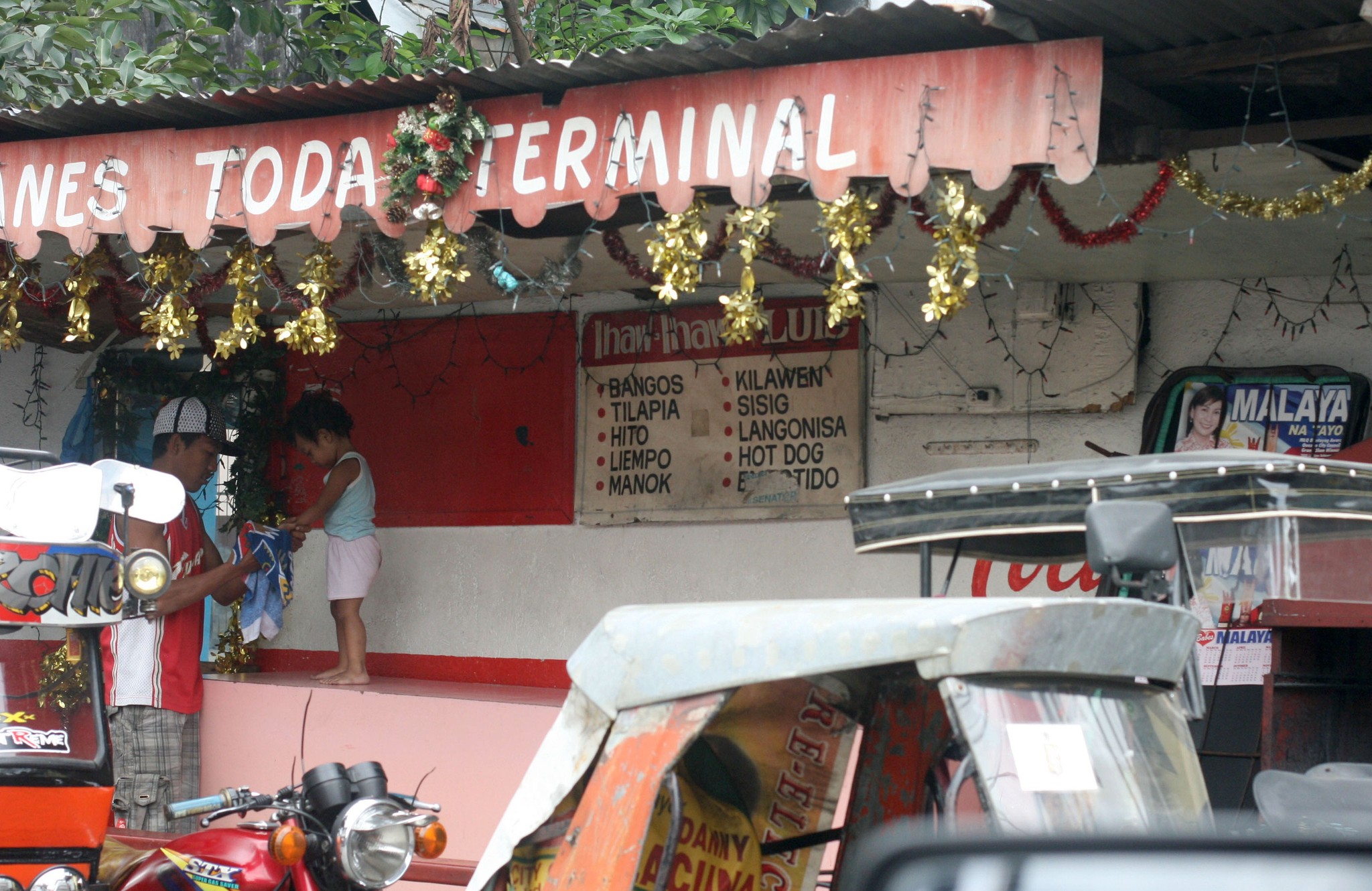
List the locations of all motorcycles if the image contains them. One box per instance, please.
[95,760,447,890]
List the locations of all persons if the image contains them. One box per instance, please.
[284,390,382,685]
[100,395,311,835]
[1176,387,1233,452]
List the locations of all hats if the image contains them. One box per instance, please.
[152,395,246,457]
